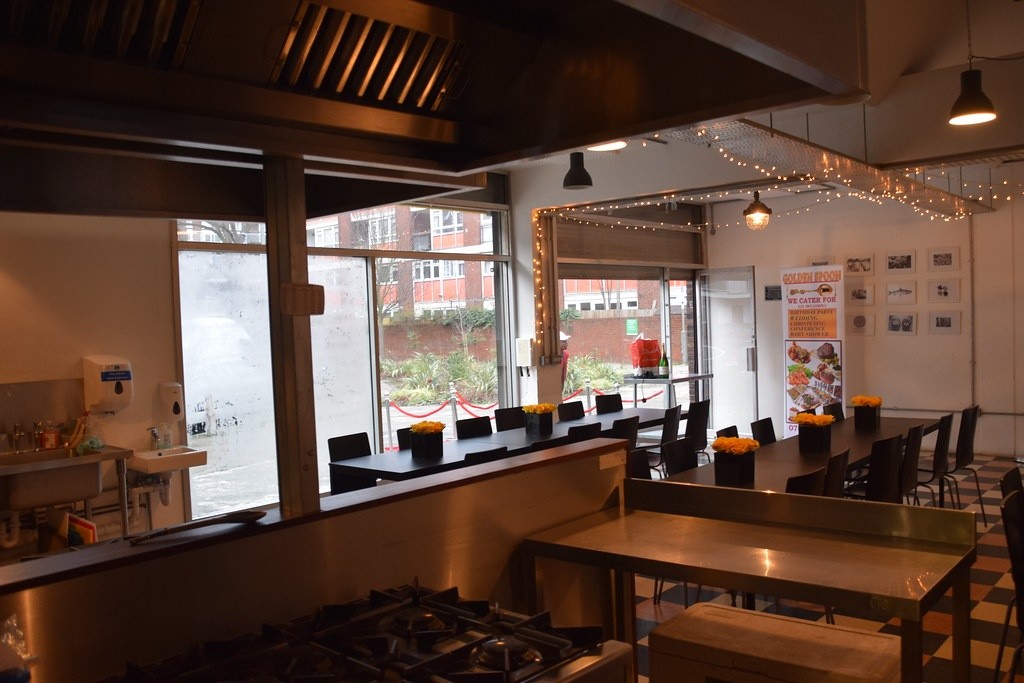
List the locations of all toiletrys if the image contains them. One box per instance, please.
[157,422,173,450]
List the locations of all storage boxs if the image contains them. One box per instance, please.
[648,602,901,683]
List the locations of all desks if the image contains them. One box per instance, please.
[328,441,529,495]
[559,407,690,438]
[663,436,873,493]
[830,415,939,447]
[456,422,614,453]
[525,478,978,683]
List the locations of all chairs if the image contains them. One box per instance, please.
[327,394,776,479]
[786,400,1024,683]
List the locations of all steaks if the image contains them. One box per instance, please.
[816,342,834,358]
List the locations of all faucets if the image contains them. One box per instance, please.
[145,426,161,451]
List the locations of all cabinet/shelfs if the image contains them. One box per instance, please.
[625,373,713,445]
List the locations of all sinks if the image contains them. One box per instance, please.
[126,444,208,474]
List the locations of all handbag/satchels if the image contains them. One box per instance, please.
[630,332,662,377]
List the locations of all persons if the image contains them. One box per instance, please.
[559,331,572,392]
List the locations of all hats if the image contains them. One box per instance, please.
[560,331,572,341]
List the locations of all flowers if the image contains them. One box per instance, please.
[520,402,557,415]
[792,413,836,427]
[849,395,882,408]
[712,436,760,455]
[409,421,445,435]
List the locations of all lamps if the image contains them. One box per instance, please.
[743,190,773,231]
[562,152,593,190]
[948,0,997,125]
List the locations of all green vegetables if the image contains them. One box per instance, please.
[788,363,812,379]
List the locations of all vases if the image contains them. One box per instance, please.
[410,433,444,459]
[524,413,553,436]
[714,452,756,489]
[854,407,880,431]
[798,424,831,453]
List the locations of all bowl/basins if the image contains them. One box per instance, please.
[60,433,87,447]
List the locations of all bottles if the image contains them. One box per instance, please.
[659,343,669,378]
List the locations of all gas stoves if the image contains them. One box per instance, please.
[93,576,603,683]
